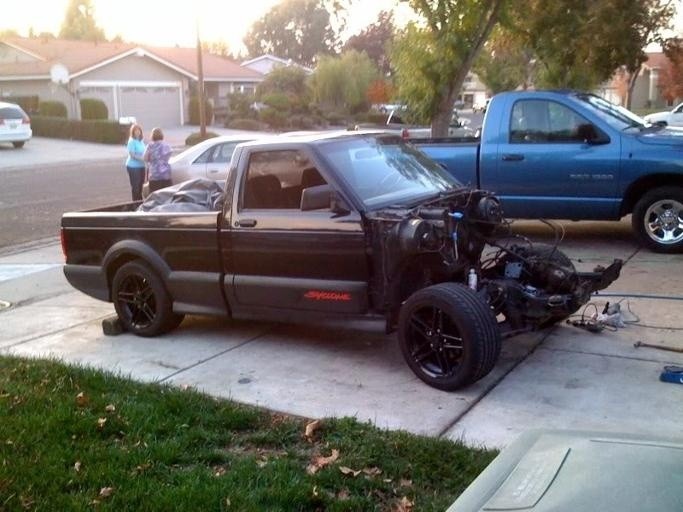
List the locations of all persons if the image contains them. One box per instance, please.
[142,126,172,193]
[124,123,147,200]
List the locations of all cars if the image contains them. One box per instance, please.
[452,100,464,109]
[0,101,32,148]
[378,102,408,114]
[644,101,682,128]
[141,132,315,198]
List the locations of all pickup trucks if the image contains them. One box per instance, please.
[345,88,682,255]
[354,106,474,141]
[59,129,624,395]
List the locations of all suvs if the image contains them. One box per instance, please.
[472,98,489,114]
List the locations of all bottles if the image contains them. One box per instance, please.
[402,128,409,141]
